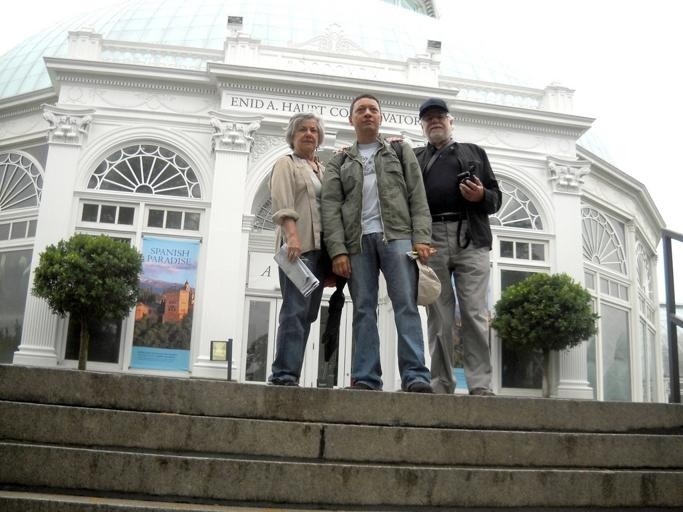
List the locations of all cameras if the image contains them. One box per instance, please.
[456,171,474,187]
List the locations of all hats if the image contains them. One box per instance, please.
[415,260,442,305]
[419,98,448,118]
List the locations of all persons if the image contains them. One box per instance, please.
[321,94,434,393]
[269,112,325,387]
[386,98,502,396]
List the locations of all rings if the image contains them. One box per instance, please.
[290,250,295,256]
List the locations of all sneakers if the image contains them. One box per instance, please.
[268,374,298,386]
[472,388,495,395]
[345,383,371,389]
[409,383,433,392]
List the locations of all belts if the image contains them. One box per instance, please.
[432,213,468,222]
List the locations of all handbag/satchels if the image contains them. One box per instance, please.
[324,270,337,286]
[469,213,492,248]
